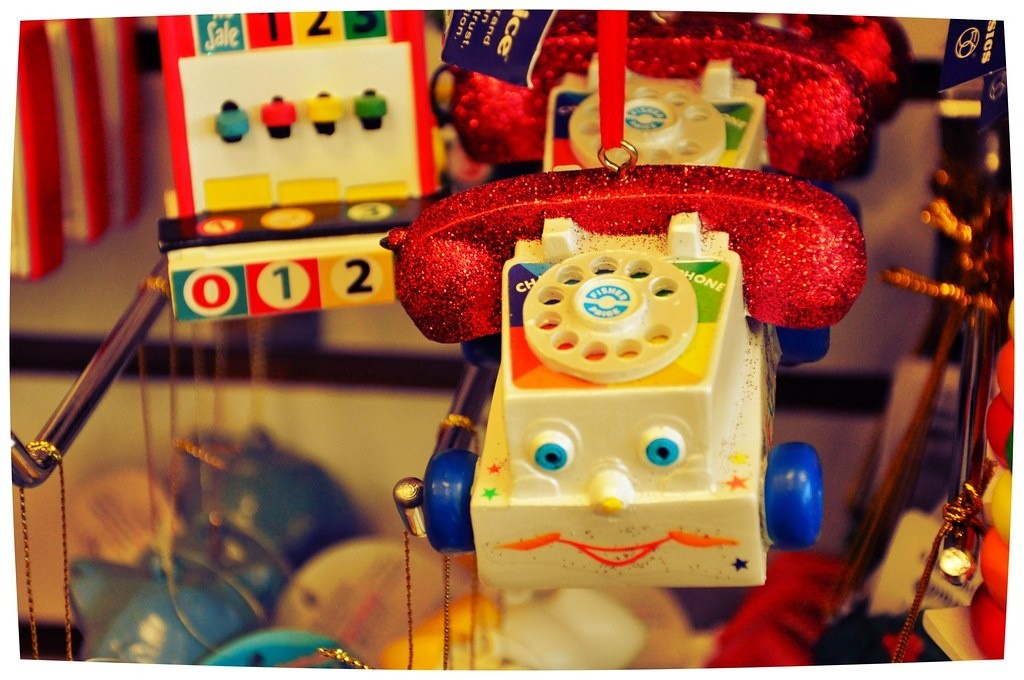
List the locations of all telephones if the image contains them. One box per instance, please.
[450,9,911,179]
[381,165,867,588]
